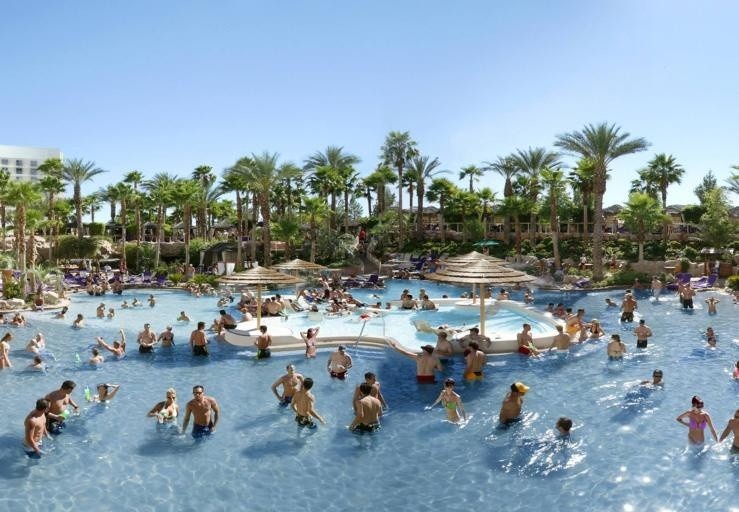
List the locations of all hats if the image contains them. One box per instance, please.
[468,327,480,333]
[445,378,455,386]
[511,382,530,393]
[420,344,434,354]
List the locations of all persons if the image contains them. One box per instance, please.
[0,254,738,461]
[496,224,505,241]
[359,229,365,241]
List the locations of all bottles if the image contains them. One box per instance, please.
[74,352,79,362]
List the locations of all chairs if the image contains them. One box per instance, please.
[281,291,356,323]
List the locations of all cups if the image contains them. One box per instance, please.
[84,389,90,401]
[529,343,532,348]
[62,409,69,418]
[159,417,163,424]
